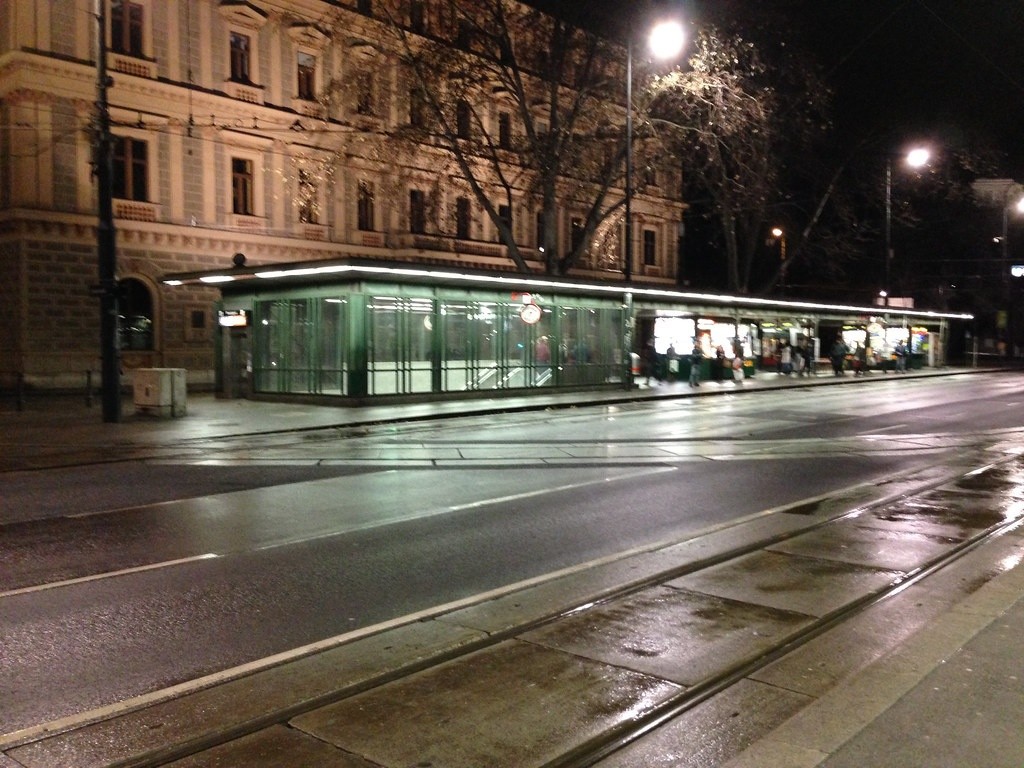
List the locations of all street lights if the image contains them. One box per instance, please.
[771,228,786,262]
[623,21,688,394]
[1001,198,1024,292]
[886,146,933,298]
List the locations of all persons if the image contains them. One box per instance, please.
[537,336,551,364]
[642,339,751,387]
[775,338,816,377]
[828,337,907,377]
